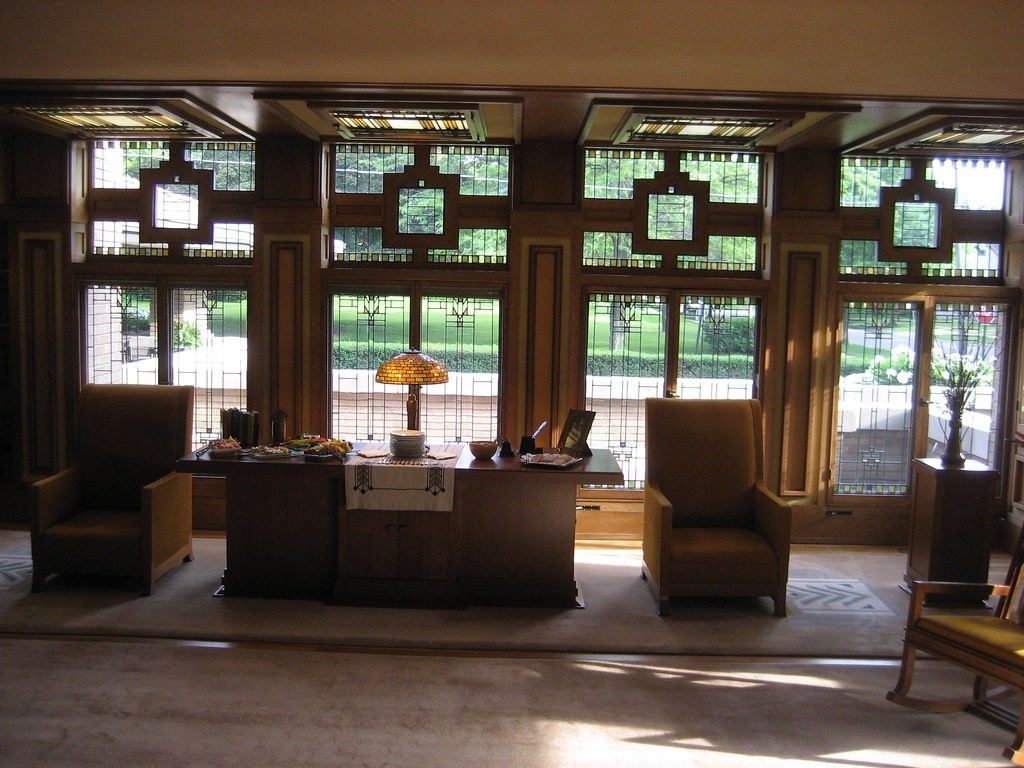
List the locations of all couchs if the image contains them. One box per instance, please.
[27,383,195,597]
[641,397,791,618]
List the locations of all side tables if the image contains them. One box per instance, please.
[897,457,1000,610]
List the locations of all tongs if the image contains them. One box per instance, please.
[329,448,344,464]
[196,443,218,457]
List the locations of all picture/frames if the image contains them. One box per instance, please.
[555,409,597,463]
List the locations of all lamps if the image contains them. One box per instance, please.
[375,347,448,430]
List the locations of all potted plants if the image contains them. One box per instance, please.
[918,352,998,465]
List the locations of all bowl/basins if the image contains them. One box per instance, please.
[470,441,497,460]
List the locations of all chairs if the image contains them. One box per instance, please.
[886,524,1024,767]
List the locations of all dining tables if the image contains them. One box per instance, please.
[175,438,624,610]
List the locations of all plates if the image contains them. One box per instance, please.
[300,452,345,461]
[521,453,577,468]
[210,447,242,457]
[390,429,426,458]
[252,452,291,459]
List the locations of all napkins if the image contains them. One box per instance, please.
[422,449,457,460]
[355,449,391,458]
